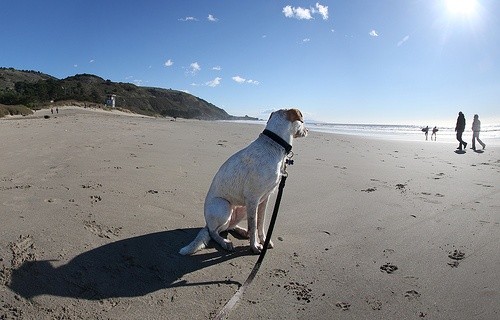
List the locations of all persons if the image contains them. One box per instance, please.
[455,112,467,149]
[425,126,436,135]
[470,115,485,149]
[51,107,58,114]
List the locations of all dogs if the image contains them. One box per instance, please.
[180,108,308,257]
